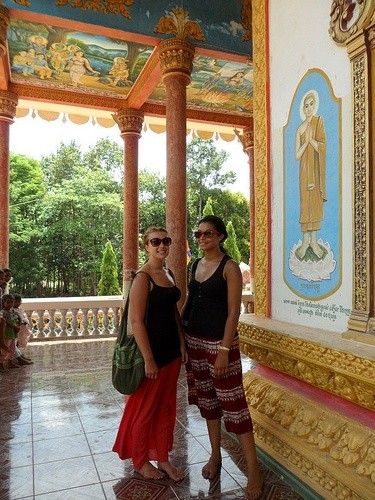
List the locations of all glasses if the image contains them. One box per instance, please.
[195,229,219,238]
[146,237,171,247]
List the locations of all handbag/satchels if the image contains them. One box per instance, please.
[112,334,144,394]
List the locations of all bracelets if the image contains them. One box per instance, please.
[218,346,229,351]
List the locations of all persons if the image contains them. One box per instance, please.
[180,215,263,500]
[0,294,21,372]
[10,294,32,355]
[112,227,188,481]
[0,268,34,366]
